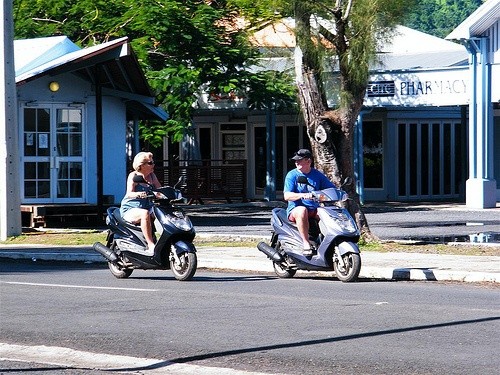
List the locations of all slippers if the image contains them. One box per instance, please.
[294,151,310,156]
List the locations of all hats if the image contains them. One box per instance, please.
[291,149,311,160]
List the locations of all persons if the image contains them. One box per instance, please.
[119,152,166,255]
[283,149,348,256]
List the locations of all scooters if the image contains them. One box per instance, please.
[257,176,362,283]
[93,175,197,281]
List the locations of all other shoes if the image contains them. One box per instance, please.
[148,244,155,254]
[303,244,315,251]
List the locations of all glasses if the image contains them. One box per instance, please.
[141,161,156,165]
